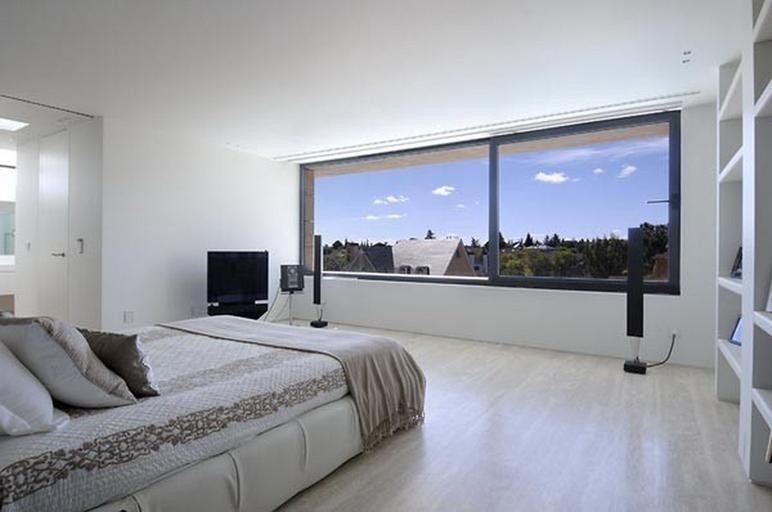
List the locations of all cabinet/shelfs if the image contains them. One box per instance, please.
[39,117,103,332]
[713,1,772,487]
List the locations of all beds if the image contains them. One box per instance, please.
[0,315,426,512]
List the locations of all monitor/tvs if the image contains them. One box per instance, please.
[206,251,269,304]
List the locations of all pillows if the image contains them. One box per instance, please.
[0,311,161,435]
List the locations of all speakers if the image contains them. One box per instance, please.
[626,227,643,338]
[314,235,322,305]
